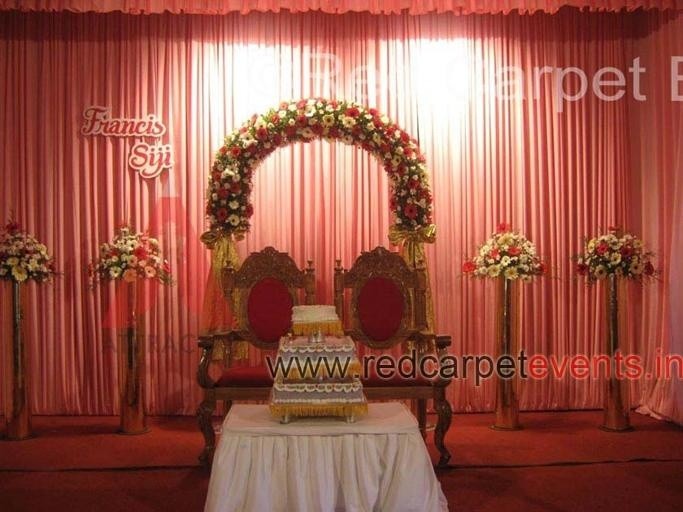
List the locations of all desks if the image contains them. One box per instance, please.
[203,403,450,512]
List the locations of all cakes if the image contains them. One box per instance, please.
[268,304,365,404]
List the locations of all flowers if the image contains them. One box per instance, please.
[0,209,64,287]
[571,226,665,286]
[457,222,561,284]
[206,97,433,232]
[84,223,177,288]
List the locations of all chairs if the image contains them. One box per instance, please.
[196,247,315,467]
[333,247,451,469]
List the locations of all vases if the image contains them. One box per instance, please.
[118,281,149,434]
[5,282,32,440]
[491,280,522,431]
[601,278,633,432]
